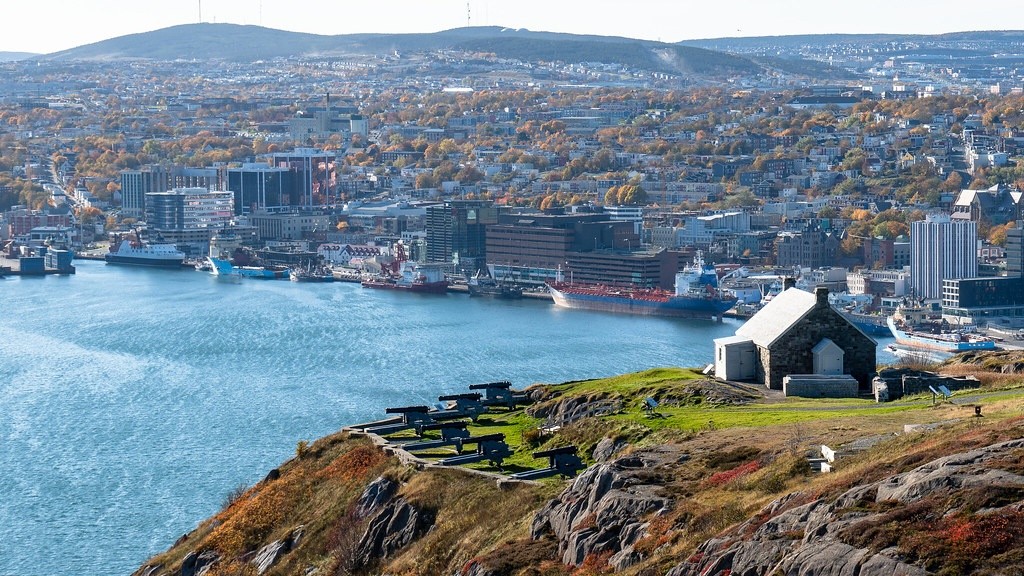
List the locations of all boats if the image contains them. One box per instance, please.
[886,286,995,353]
[544,247,739,318]
[466,270,552,300]
[207,248,382,282]
[359,239,449,293]
[105,224,185,266]
